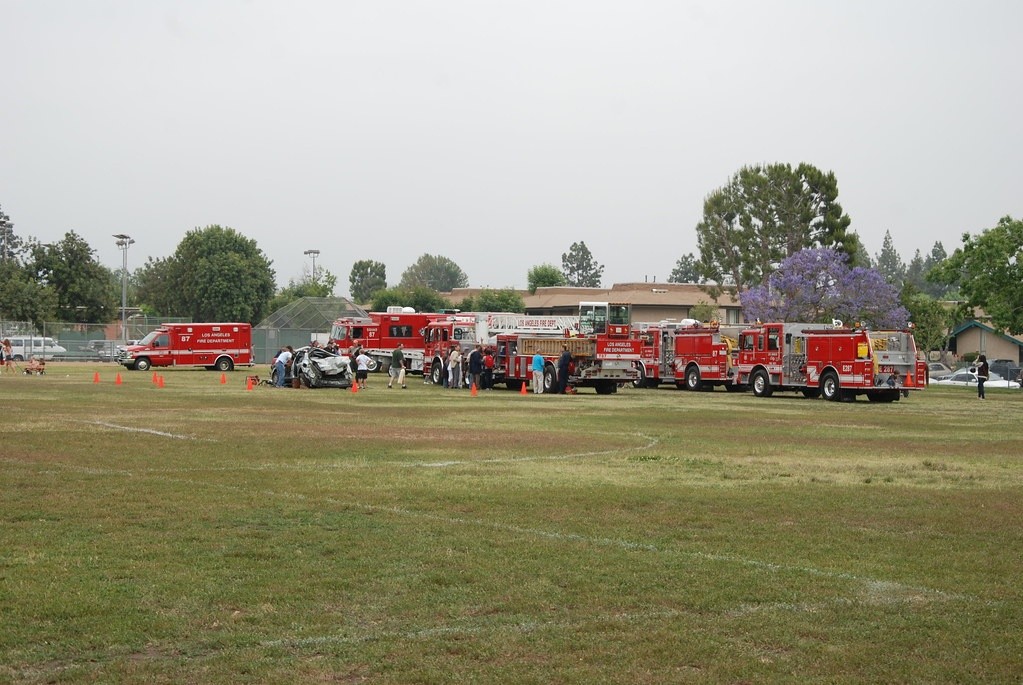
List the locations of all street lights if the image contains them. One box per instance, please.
[304,250,320,281]
[113,234,135,339]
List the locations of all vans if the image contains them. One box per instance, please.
[2,337,69,362]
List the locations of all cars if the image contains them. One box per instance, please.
[928,359,1023,385]
[939,373,1021,389]
[271,346,354,389]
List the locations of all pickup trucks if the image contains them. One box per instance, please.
[79,341,121,361]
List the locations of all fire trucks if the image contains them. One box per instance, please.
[736,317,926,404]
[631,318,754,393]
[422,300,641,395]
[328,306,516,373]
[116,323,255,372]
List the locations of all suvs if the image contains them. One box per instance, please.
[127,340,141,345]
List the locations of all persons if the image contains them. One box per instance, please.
[972,355,989,400]
[442,343,495,392]
[387,342,407,389]
[886,370,900,388]
[556,345,570,395]
[28,356,45,372]
[0,340,3,365]
[308,338,372,389]
[3,339,16,376]
[272,345,294,389]
[531,348,545,394]
[391,328,410,337]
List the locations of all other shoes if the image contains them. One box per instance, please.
[388,385,392,389]
[424,382,429,384]
[402,385,407,389]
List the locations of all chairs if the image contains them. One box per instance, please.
[769,338,779,350]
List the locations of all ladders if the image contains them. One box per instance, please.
[521,337,593,357]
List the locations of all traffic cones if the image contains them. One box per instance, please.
[352,379,357,392]
[220,374,253,390]
[471,381,527,395]
[904,370,914,388]
[92,371,99,383]
[116,373,122,384]
[151,372,164,388]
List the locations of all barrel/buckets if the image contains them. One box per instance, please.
[292,377,300,388]
